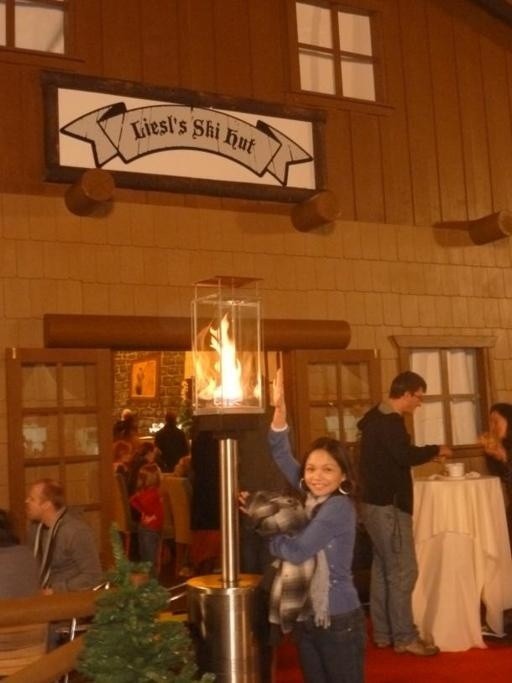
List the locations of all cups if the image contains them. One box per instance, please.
[448,462,464,477]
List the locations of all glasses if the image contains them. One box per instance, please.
[413,394,424,401]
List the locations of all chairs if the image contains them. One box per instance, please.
[1,565,116,683]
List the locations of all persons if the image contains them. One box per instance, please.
[113,408,136,441]
[156,411,188,473]
[24,479,103,650]
[130,462,164,580]
[357,371,452,657]
[174,456,196,486]
[127,443,161,521]
[0,511,41,600]
[481,403,512,638]
[238,370,365,683]
[192,431,221,576]
[113,440,134,472]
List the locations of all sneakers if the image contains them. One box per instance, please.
[480,625,507,639]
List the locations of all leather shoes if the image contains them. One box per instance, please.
[376,638,438,656]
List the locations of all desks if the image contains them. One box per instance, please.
[412,473,511,652]
